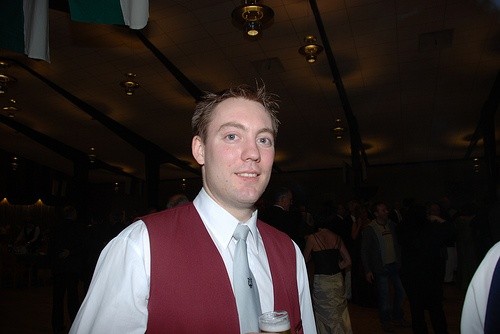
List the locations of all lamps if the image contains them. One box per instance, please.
[121,73,142,97]
[331,117,346,140]
[297,31,324,64]
[230,0,275,42]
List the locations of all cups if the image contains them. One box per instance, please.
[258,310,291,334]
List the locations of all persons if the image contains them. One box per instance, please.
[160,192,189,208]
[14,217,40,286]
[303,206,353,334]
[427,203,499,285]
[68,85,317,334]
[297,200,375,282]
[360,202,410,329]
[395,198,447,334]
[47,204,86,334]
[263,186,297,244]
